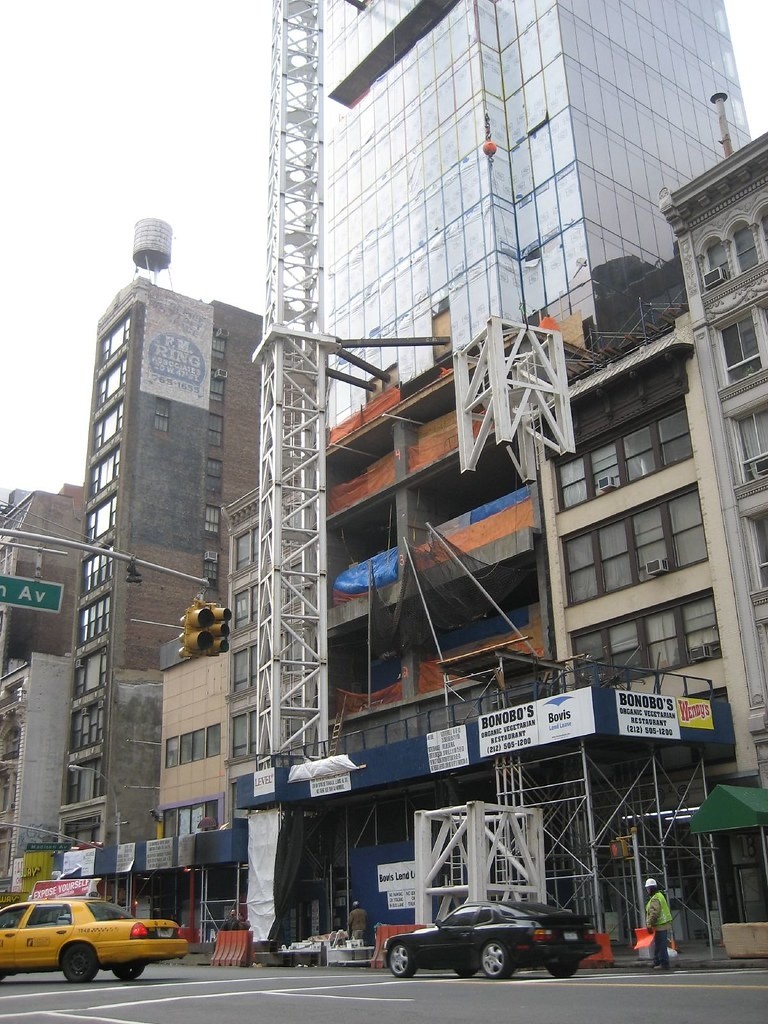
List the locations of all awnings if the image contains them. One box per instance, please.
[689,784,768,959]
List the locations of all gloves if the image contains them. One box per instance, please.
[647,927,653,934]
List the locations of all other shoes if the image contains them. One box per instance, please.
[654,963,670,971]
[647,961,659,968]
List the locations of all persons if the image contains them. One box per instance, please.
[348,901,368,940]
[222,909,244,930]
[644,879,673,971]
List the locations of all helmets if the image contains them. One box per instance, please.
[645,878,657,888]
[230,910,236,915]
[351,901,361,906]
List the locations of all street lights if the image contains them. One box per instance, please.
[69,763,120,907]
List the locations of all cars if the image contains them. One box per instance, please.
[383,901,603,980]
[0,878,190,984]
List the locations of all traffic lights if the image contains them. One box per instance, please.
[206,604,233,656]
[178,608,190,658]
[186,607,214,656]
